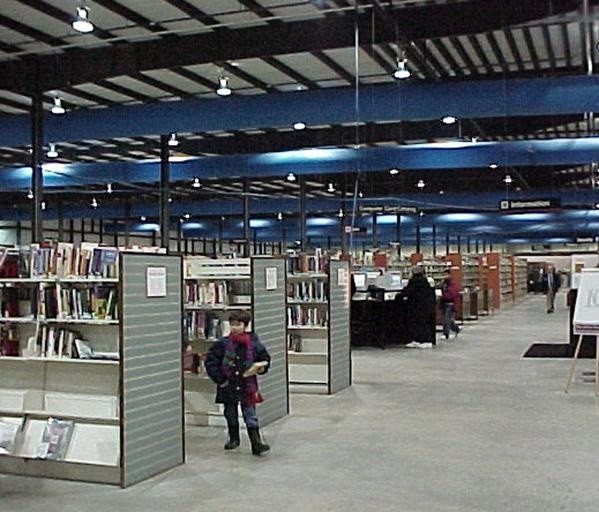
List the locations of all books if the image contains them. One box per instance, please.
[36,418,75,461]
[242,361,267,378]
[0,243,121,360]
[464,255,531,320]
[287,279,328,352]
[183,281,228,341]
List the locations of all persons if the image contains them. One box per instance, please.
[351,270,357,306]
[204,308,271,454]
[438,270,462,342]
[397,267,435,348]
[540,265,558,315]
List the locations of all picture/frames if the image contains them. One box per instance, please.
[571,265,599,327]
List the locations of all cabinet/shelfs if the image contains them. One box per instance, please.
[180,251,293,432]
[353,249,532,350]
[278,251,353,403]
[0,242,187,494]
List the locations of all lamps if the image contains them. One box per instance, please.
[214,73,236,100]
[41,201,45,210]
[194,179,200,187]
[287,173,295,181]
[50,95,66,115]
[107,183,112,193]
[392,55,412,79]
[70,3,97,36]
[28,189,34,199]
[46,143,58,157]
[92,198,97,207]
[168,134,178,145]
[168,198,171,202]
[328,183,336,192]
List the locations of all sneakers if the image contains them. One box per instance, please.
[416,342,433,349]
[405,340,421,348]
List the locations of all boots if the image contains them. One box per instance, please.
[224,424,240,450]
[248,426,270,455]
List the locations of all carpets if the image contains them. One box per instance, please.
[522,338,570,359]
